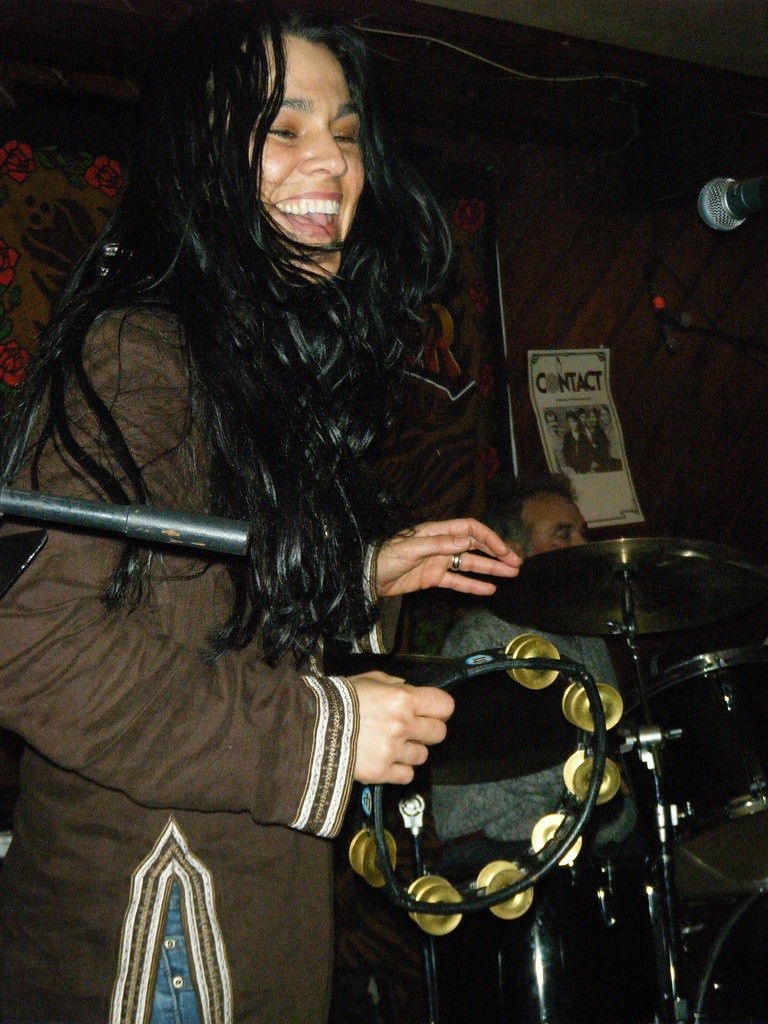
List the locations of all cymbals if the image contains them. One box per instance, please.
[481,537,768,639]
[343,635,622,937]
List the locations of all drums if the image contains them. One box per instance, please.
[620,645,768,894]
[655,893,768,1024]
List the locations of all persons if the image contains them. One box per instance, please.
[431,473,640,865]
[545,409,617,472]
[1,2,528,1023]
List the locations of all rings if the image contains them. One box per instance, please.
[452,553,460,569]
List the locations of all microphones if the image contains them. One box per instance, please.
[697,176,768,232]
